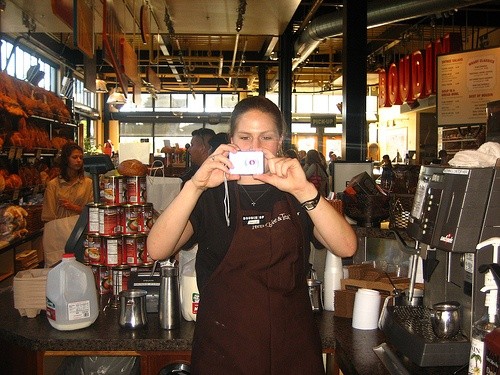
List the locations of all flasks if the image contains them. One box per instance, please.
[158,266,181,330]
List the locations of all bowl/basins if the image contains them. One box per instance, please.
[337,192,390,228]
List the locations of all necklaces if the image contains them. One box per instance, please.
[237,180,272,207]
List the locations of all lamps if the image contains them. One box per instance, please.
[106,75,126,104]
[96,72,108,94]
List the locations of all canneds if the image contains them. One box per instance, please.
[83,175,154,296]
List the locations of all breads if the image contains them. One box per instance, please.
[0,72,71,242]
[117,159,147,176]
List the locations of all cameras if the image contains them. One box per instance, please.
[228,150,265,174]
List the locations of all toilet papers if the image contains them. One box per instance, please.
[353,331,381,364]
[351,288,382,330]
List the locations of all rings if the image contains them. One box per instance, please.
[211,156,214,161]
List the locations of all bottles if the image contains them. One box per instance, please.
[307,279,322,311]
[45,253,99,331]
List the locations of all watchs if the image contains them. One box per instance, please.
[300,191,321,211]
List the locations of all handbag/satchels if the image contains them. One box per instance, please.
[309,165,321,190]
[145,161,182,212]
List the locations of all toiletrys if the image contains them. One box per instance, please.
[469,236,500,375]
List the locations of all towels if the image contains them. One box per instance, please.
[448,141,500,168]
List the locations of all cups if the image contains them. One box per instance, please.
[392,287,424,306]
[431,302,461,340]
[322,250,348,312]
[352,288,381,330]
[117,289,148,329]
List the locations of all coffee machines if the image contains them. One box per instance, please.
[382,163,500,369]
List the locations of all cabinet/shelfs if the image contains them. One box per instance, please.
[0,71,78,298]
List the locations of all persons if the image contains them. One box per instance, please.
[181,128,230,189]
[147,96,358,375]
[285,144,447,197]
[40,142,93,267]
